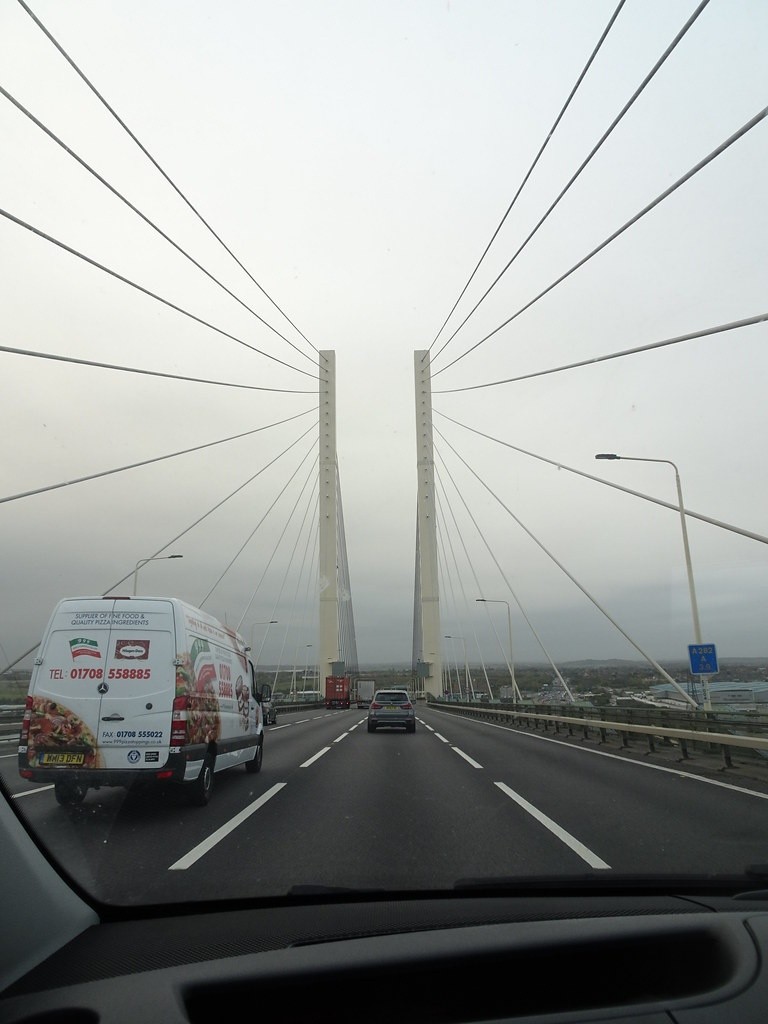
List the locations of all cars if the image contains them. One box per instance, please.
[367,689,416,734]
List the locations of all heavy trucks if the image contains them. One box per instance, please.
[325,676,352,710]
[357,680,375,709]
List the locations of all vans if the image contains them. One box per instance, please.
[16,594,273,807]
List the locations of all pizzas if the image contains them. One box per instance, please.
[28,696,106,768]
[175,653,222,746]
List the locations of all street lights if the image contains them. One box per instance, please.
[251,620,278,652]
[133,554,184,596]
[406,649,441,701]
[596,454,713,712]
[293,644,312,699]
[445,636,469,702]
[475,599,516,705]
